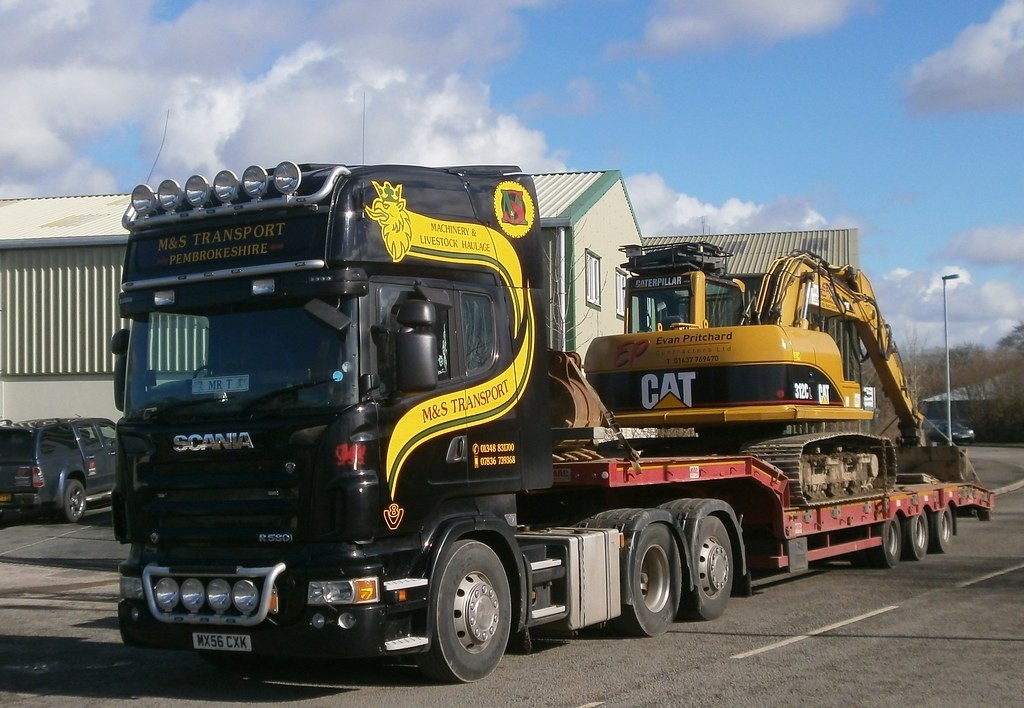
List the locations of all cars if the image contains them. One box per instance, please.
[923,418,975,443]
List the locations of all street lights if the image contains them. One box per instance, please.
[939,273,962,448]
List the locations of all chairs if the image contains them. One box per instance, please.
[80,429,92,446]
[662,315,685,331]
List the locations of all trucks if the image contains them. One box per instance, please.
[104,153,995,686]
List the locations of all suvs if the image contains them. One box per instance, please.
[0,414,118,524]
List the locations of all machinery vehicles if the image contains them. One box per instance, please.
[579,239,979,506]
